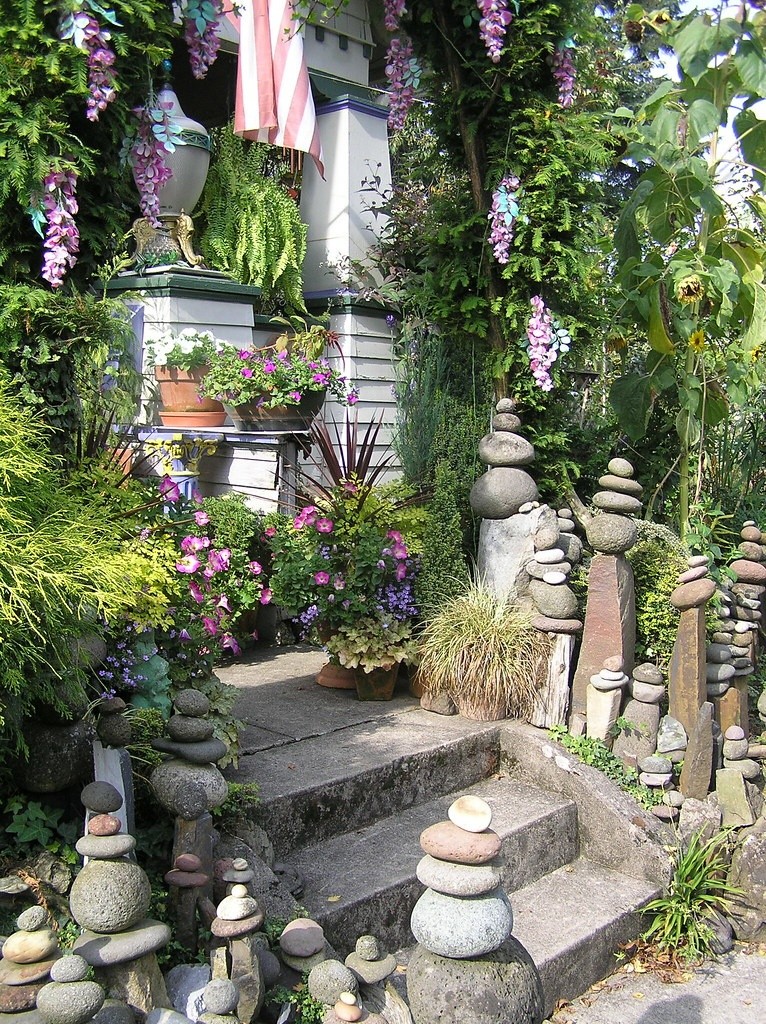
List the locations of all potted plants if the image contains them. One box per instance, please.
[416,552,552,720]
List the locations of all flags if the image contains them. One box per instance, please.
[222,0,327,182]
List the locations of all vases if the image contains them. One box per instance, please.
[151,364,226,412]
[352,663,400,701]
[314,619,358,689]
[407,666,424,699]
[222,388,327,431]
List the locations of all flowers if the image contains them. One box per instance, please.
[194,342,361,412]
[143,314,234,372]
[263,470,417,671]
